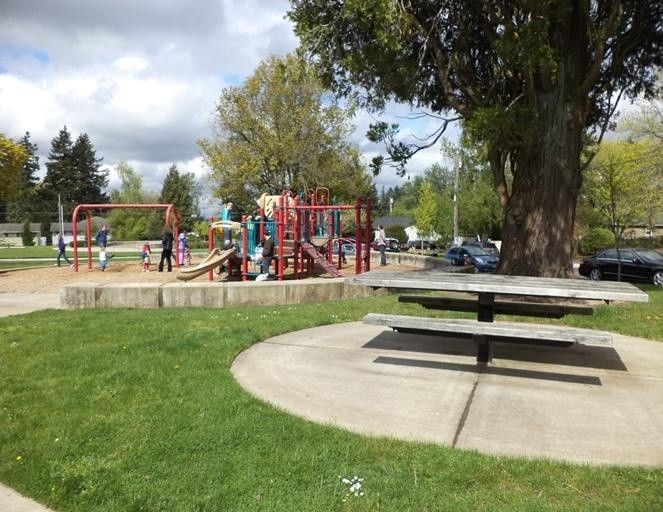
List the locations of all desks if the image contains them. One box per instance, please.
[345,270,649,363]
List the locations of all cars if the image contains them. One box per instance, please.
[333,235,437,258]
[446,246,500,272]
[579,245,663,287]
[462,240,499,257]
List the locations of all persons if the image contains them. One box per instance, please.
[155,224,174,272]
[99,244,112,272]
[140,240,151,264]
[53,234,75,268]
[375,223,388,266]
[184,244,192,266]
[142,246,151,273]
[249,240,265,263]
[261,230,274,275]
[338,241,347,264]
[220,202,234,245]
[96,223,108,266]
[178,229,188,265]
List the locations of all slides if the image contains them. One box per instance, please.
[175,247,237,281]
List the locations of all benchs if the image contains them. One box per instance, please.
[363,311,578,344]
[398,294,594,319]
